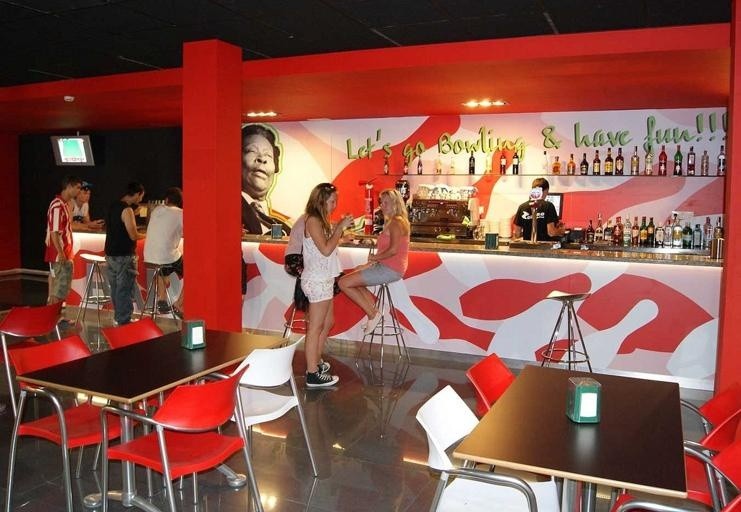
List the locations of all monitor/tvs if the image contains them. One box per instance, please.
[545,193,563,219]
[50,135,97,167]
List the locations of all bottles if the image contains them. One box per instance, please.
[384,157,390,175]
[451,156,456,174]
[486,149,492,174]
[417,154,422,176]
[584,211,726,260]
[561,228,584,245]
[435,154,444,175]
[147,199,165,217]
[543,151,549,175]
[501,151,507,175]
[404,156,410,176]
[513,153,520,174]
[469,151,476,175]
[551,144,726,177]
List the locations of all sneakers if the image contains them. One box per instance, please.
[361,316,385,328]
[305,358,331,376]
[364,312,382,336]
[305,370,339,387]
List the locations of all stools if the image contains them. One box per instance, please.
[353,277,412,368]
[279,274,309,348]
[75,253,112,328]
[140,264,179,331]
[541,293,598,372]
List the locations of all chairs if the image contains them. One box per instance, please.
[612,385,741,508]
[0,299,63,419]
[100,366,264,512]
[466,354,516,416]
[611,435,741,512]
[100,316,195,491]
[206,336,318,495]
[416,385,572,512]
[6,337,137,512]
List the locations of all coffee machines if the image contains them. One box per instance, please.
[408,199,480,241]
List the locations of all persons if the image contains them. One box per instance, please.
[338,188,411,334]
[301,182,354,388]
[70,181,105,232]
[44,179,81,325]
[285,212,345,311]
[241,125,292,236]
[513,178,565,246]
[143,187,183,320]
[241,223,247,307]
[104,187,147,328]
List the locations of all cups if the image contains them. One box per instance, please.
[477,214,513,239]
[272,224,284,239]
[344,214,355,230]
[415,182,480,201]
[485,233,500,250]
